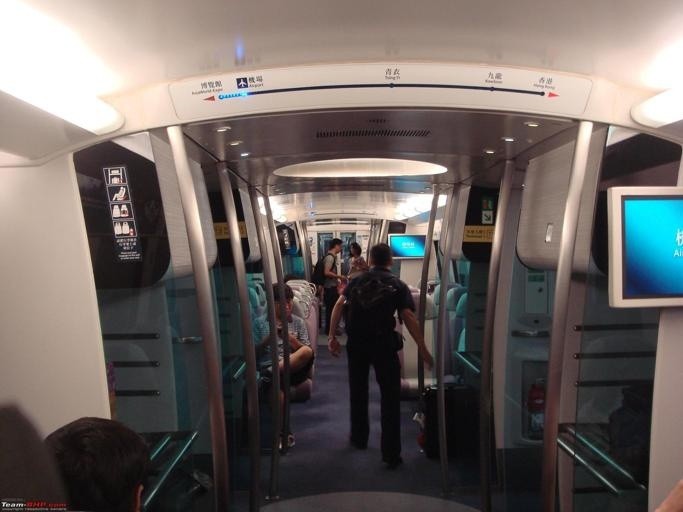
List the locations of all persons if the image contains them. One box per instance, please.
[322,238,348,338]
[345,240,370,284]
[250,281,315,453]
[42,415,152,512]
[324,241,433,473]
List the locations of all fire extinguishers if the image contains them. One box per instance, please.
[527,378,546,433]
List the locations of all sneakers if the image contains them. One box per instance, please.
[278,432,296,451]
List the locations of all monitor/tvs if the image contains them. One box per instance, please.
[388,234,426,260]
[607,186,683,309]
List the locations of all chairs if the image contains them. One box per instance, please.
[248,278,319,403]
[393,278,470,400]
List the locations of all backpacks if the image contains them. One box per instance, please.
[311,253,335,286]
[344,279,399,337]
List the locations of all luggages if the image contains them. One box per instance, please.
[423,384,474,458]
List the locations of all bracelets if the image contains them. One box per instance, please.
[327,336,337,340]
